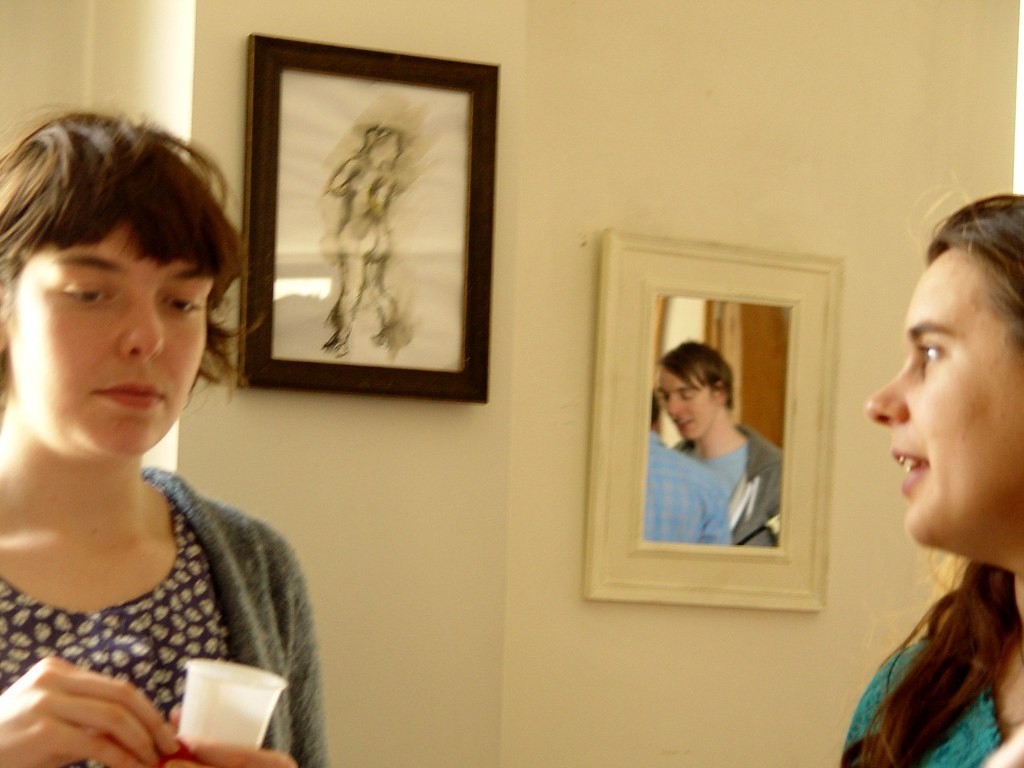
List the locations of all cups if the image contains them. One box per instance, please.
[174,657,287,751]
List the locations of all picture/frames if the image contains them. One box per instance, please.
[569,215,850,620]
[234,30,505,410]
